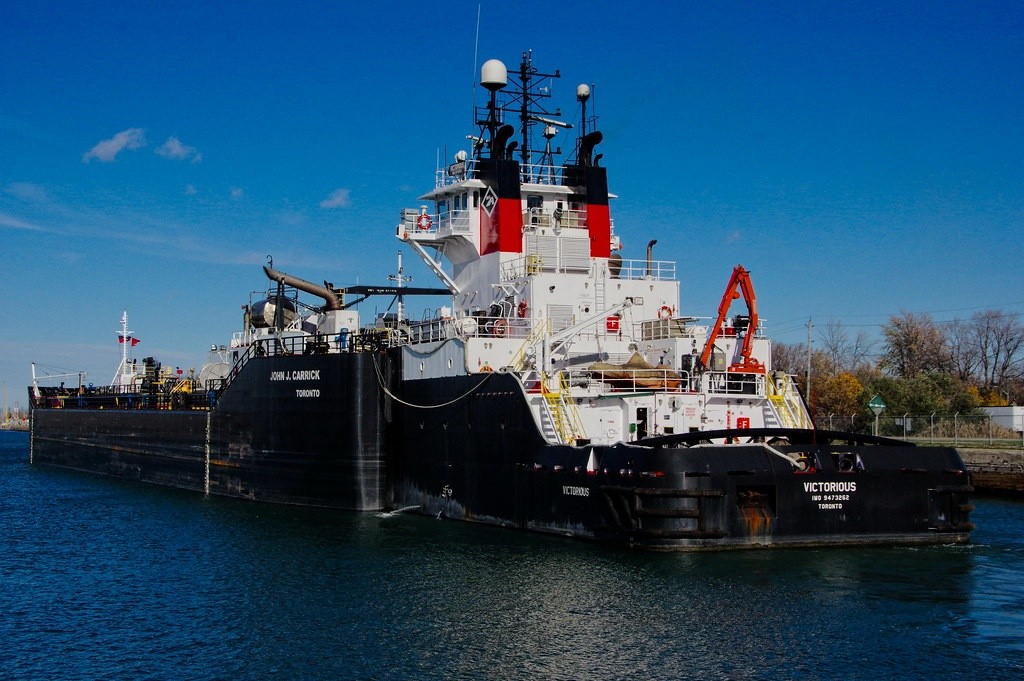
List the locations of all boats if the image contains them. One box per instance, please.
[0,380,29,431]
[26,46,977,556]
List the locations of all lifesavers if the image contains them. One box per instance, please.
[517,303,526,318]
[493,320,512,338]
[416,215,432,230]
[657,305,672,320]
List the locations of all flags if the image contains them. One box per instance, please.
[117,335,131,343]
